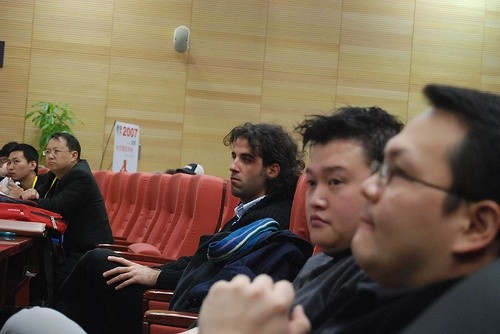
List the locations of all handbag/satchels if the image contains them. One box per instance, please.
[0,202,68,233]
[0,194,38,207]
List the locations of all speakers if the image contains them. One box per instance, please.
[173,24,190,53]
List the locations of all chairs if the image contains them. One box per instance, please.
[90,171,323,334]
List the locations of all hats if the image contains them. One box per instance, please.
[176,163,205,175]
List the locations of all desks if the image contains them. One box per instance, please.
[0,230,49,318]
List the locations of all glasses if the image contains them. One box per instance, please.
[43,149,72,155]
[370,160,477,203]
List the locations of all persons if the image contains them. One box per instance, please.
[166,163,204,175]
[53,121,306,334]
[0,132,113,309]
[0,82,500,334]
[291,107,406,320]
